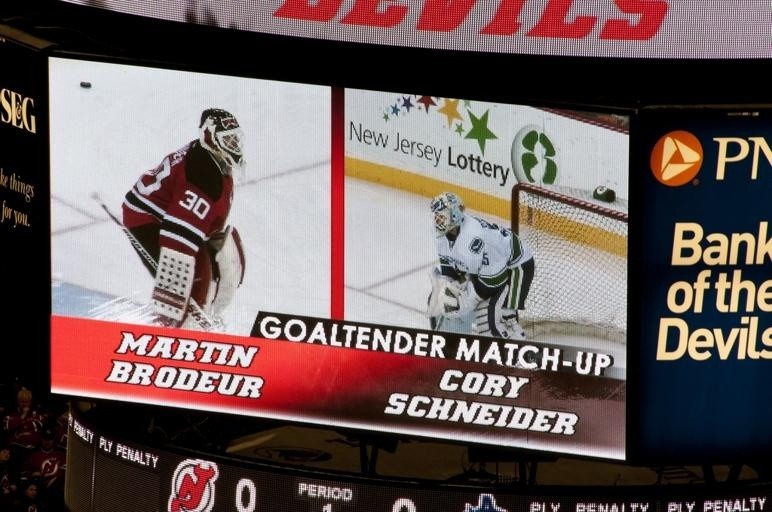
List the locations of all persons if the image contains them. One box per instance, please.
[1,381,100,512]
[121,106,248,334]
[425,190,538,341]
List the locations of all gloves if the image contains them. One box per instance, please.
[438,277,482,319]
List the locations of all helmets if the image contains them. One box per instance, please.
[199,108,247,168]
[430,192,467,239]
